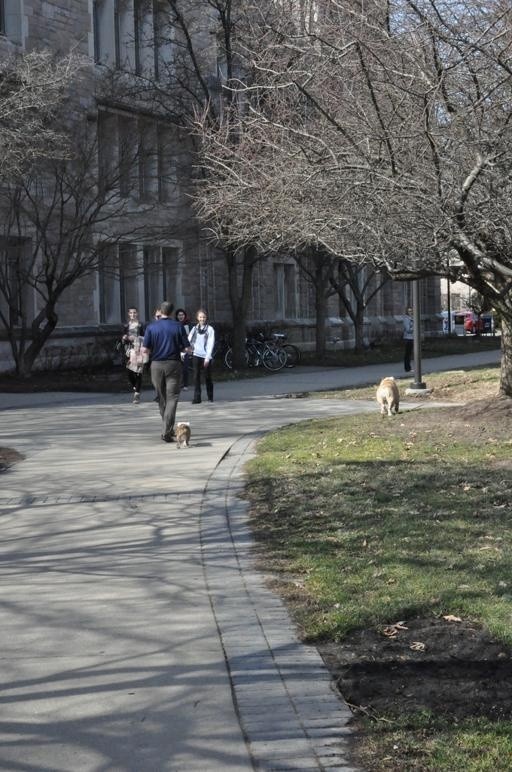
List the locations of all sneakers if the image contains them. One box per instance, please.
[162,432,176,442]
[128,384,140,404]
[192,396,213,404]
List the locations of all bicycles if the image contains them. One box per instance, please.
[224,332,303,372]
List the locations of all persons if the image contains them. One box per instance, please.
[180,308,217,404]
[402,306,414,373]
[174,309,197,390]
[117,306,148,405]
[142,301,195,443]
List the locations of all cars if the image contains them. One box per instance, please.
[479,317,498,334]
[456,312,484,334]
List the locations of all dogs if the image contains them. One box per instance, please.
[174,421,191,449]
[373,376,401,416]
